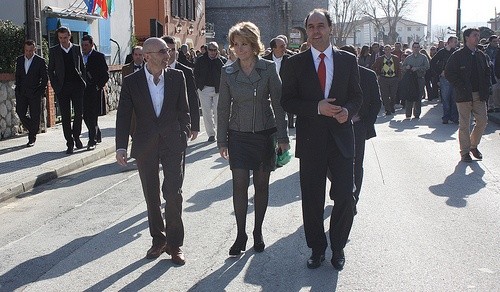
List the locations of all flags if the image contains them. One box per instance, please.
[83,0,115,19]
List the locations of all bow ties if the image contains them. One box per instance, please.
[134,65,142,70]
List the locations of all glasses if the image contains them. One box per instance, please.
[208,49,218,52]
[144,48,172,55]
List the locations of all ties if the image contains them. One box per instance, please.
[317,53,326,97]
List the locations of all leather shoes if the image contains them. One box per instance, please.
[307,254,326,268]
[331,249,345,269]
[146,241,168,258]
[165,243,185,264]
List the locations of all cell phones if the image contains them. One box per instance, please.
[278,148,282,154]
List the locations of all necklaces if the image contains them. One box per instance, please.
[241,62,254,70]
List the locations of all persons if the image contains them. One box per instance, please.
[217,22,290,256]
[121,28,500,158]
[115,36,192,265]
[47,26,87,155]
[339,45,381,216]
[280,8,363,270]
[14,40,48,147]
[81,35,109,151]
[444,29,493,163]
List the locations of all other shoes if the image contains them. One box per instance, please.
[88,142,96,150]
[470,148,483,159]
[488,109,494,112]
[451,119,459,124]
[386,110,391,115]
[391,105,395,113]
[27,140,35,146]
[495,108,500,112]
[96,137,102,142]
[442,119,448,123]
[66,147,73,155]
[208,136,215,142]
[461,153,472,162]
[73,136,82,149]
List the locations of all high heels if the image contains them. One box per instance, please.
[230,233,248,256]
[253,230,264,252]
